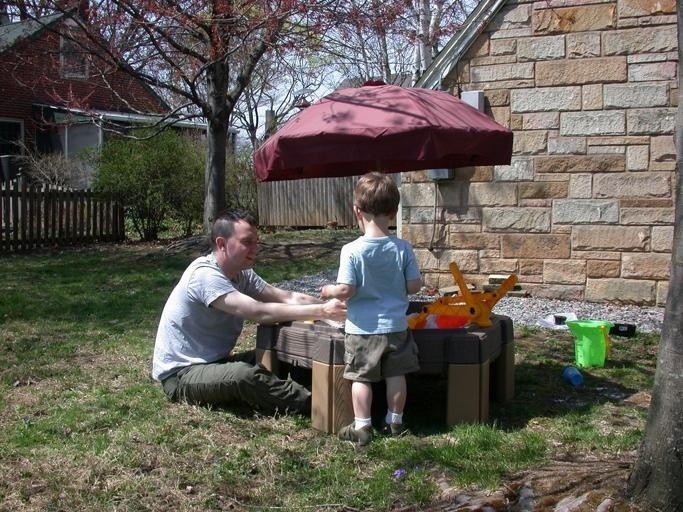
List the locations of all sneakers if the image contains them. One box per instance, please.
[338,421,374,446]
[379,420,402,436]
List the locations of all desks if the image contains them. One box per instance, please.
[252,304,515,435]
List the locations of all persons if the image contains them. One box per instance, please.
[152,208,348,413]
[320,171,422,443]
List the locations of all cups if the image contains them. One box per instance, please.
[563,366,583,386]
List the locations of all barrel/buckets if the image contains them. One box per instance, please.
[565,320,615,368]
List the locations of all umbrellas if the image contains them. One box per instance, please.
[253,78,514,184]
